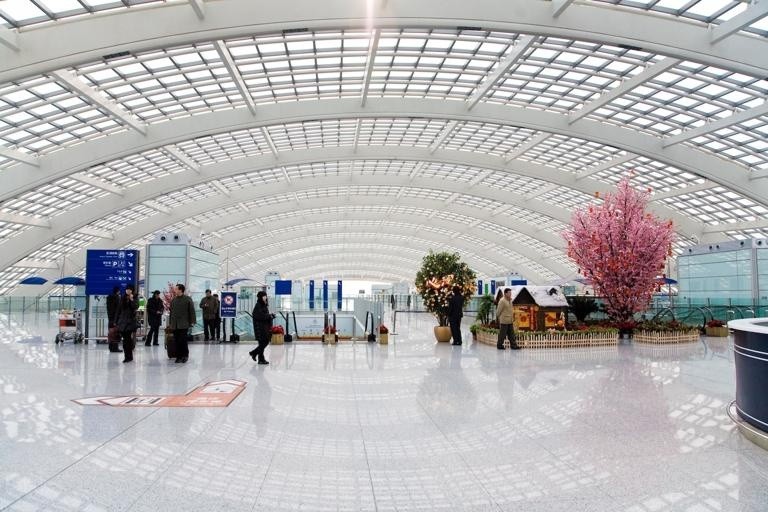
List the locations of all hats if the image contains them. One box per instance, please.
[257,291,266,297]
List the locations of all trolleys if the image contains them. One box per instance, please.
[55,309,82,344]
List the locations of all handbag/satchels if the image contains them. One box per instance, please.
[108,326,122,344]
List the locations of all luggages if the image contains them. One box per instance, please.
[167,328,177,358]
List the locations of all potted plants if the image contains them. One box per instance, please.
[413,249,478,342]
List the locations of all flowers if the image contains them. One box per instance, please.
[272,325,285,336]
[380,325,388,334]
[705,318,725,327]
[323,326,337,334]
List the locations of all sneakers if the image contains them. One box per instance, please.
[175,357,188,363]
[145,344,159,346]
[498,346,517,349]
[110,349,133,363]
[204,337,219,341]
[250,352,269,364]
[452,341,462,345]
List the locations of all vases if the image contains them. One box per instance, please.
[705,326,729,337]
[271,334,284,345]
[324,334,335,343]
[380,334,388,344]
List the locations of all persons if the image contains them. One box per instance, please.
[447,285,464,346]
[248,291,276,365]
[144,290,164,346]
[164,284,196,363]
[113,283,139,363]
[495,288,522,350]
[106,285,122,353]
[198,288,218,340]
[212,294,220,341]
